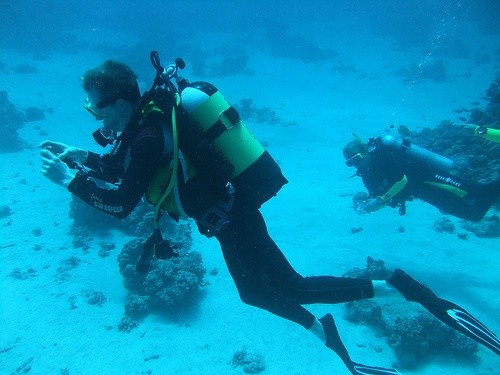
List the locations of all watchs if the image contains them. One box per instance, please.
[476,125,488,143]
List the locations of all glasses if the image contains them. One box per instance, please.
[85,82,138,120]
[346,147,369,167]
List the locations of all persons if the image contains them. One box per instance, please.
[37,58,500,375]
[343,123,500,221]
